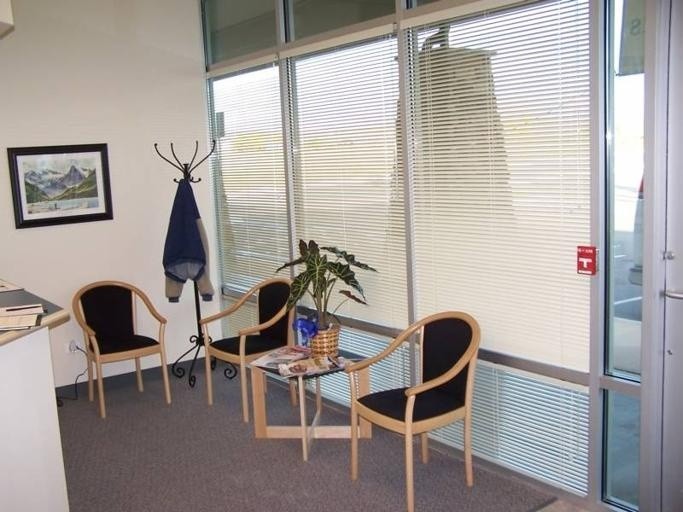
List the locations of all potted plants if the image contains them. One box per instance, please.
[277,239,381,360]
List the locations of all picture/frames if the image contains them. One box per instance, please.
[8,141,116,229]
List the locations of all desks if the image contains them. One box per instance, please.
[0,278,71,512]
[248,345,372,461]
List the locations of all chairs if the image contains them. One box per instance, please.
[198,277,299,421]
[72,281,171,418]
[343,311,481,511]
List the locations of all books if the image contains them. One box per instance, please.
[249,344,356,379]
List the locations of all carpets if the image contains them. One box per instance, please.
[56,355,559,512]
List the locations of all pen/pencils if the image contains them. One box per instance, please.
[6,305,42,312]
